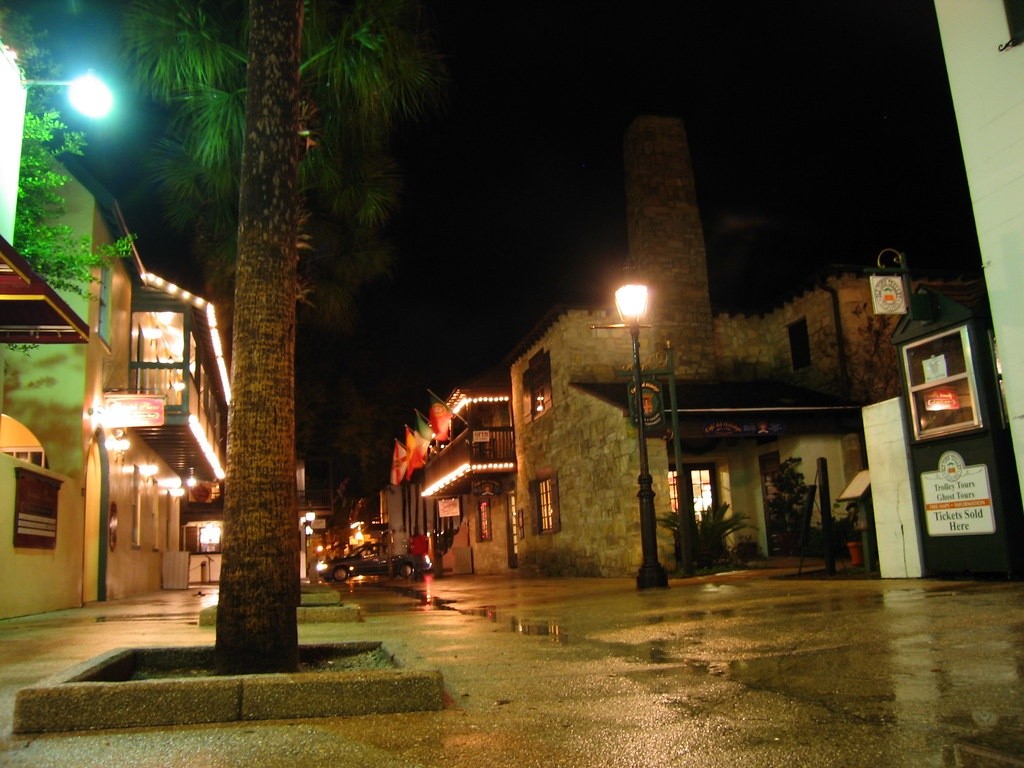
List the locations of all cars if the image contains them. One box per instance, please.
[319,542,414,582]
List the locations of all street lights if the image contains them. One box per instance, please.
[613,254,669,590]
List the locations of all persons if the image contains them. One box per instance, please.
[410,529,426,580]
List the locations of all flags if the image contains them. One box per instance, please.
[406,413,432,481]
[430,394,451,442]
[390,440,409,485]
